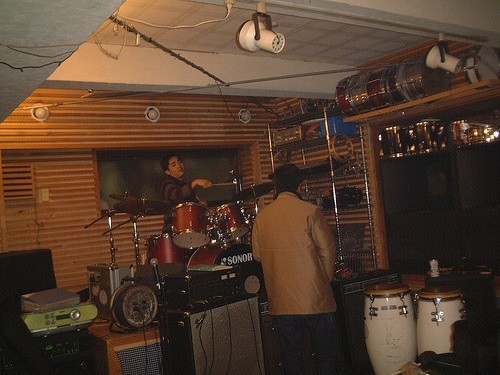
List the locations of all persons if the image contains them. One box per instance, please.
[159,153,212,234]
[251,165,342,375]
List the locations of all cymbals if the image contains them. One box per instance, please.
[114,200,171,216]
[110,193,143,201]
[329,133,354,163]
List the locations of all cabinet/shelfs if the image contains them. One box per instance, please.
[267,109,378,274]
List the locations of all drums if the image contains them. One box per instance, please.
[378,124,418,158]
[414,118,447,154]
[187,242,258,268]
[145,232,183,274]
[171,202,212,248]
[362,283,417,375]
[212,203,250,243]
[233,182,274,200]
[448,117,500,149]
[335,54,453,118]
[414,286,467,375]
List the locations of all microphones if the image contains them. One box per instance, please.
[149,257,162,289]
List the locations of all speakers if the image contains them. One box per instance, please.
[257,299,316,375]
[332,268,398,375]
[164,291,265,375]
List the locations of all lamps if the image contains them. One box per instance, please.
[237,2,286,53]
[426,32,462,73]
